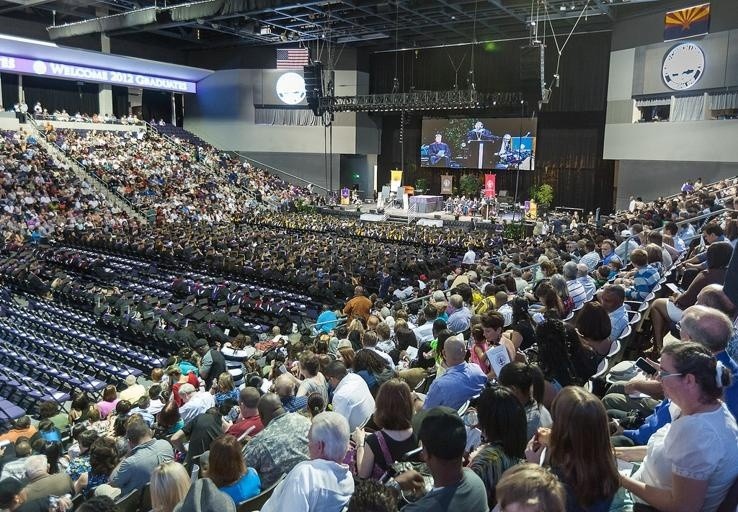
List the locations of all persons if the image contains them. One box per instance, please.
[335,346,355,370]
[68,392,100,426]
[193,338,227,392]
[636,176,738,229]
[466,121,498,162]
[495,291,513,327]
[516,319,578,387]
[243,335,256,358]
[128,396,155,429]
[353,349,396,399]
[94,413,175,503]
[38,418,63,452]
[0,436,30,473]
[499,362,553,442]
[374,322,396,354]
[610,341,738,512]
[177,347,198,376]
[413,304,443,348]
[147,461,190,512]
[601,284,738,417]
[609,259,620,278]
[289,350,329,413]
[74,437,120,495]
[215,334,252,392]
[165,365,200,412]
[326,213,488,244]
[295,328,352,350]
[640,242,738,360]
[274,374,309,413]
[343,286,372,327]
[259,411,354,512]
[474,284,499,315]
[495,462,568,512]
[148,385,168,414]
[119,375,146,406]
[396,245,450,308]
[618,244,667,283]
[40,440,71,475]
[551,273,575,315]
[347,318,365,344]
[430,318,450,363]
[383,406,489,511]
[535,281,564,321]
[525,385,622,512]
[349,330,364,349]
[576,301,611,369]
[0,440,41,483]
[428,134,452,167]
[474,234,561,290]
[601,238,624,269]
[343,245,371,286]
[85,409,110,437]
[411,336,488,415]
[662,222,686,252]
[615,248,660,303]
[371,245,396,321]
[201,434,261,510]
[68,423,88,458]
[113,415,135,457]
[107,400,132,434]
[551,206,579,259]
[38,401,68,431]
[442,195,498,218]
[394,317,418,352]
[324,360,376,432]
[581,210,637,243]
[647,230,673,269]
[97,385,121,420]
[65,429,98,481]
[347,478,400,512]
[254,323,295,387]
[562,261,586,308]
[153,400,185,442]
[23,454,75,499]
[447,265,473,293]
[353,329,394,380]
[242,244,313,330]
[0,416,37,444]
[458,284,475,314]
[304,393,326,420]
[494,133,519,169]
[628,196,635,213]
[365,316,378,333]
[465,385,527,512]
[178,383,216,424]
[677,224,733,291]
[541,220,550,236]
[315,304,338,334]
[0,477,55,512]
[480,311,516,379]
[314,244,343,303]
[242,392,313,491]
[15,101,165,127]
[461,244,476,265]
[594,265,608,287]
[224,387,264,439]
[577,262,596,302]
[213,372,240,409]
[469,323,493,374]
[171,407,224,479]
[0,244,242,347]
[637,229,679,262]
[351,377,421,480]
[601,285,629,342]
[330,184,362,206]
[395,309,417,330]
[607,305,738,448]
[148,368,168,392]
[532,218,542,236]
[509,294,538,349]
[579,240,600,274]
[446,294,471,335]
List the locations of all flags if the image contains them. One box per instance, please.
[276,48,309,71]
[663,3,711,43]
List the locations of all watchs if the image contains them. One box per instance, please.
[386,477,401,491]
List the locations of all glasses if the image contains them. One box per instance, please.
[675,321,681,332]
[658,370,681,379]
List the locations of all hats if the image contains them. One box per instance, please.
[192,339,208,349]
[0,477,29,509]
[172,478,236,512]
[411,406,467,460]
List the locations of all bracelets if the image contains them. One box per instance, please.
[356,443,364,450]
[413,397,420,402]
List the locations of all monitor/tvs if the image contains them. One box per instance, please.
[423,111,537,173]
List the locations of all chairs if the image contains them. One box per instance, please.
[186,271,313,309]
[301,319,306,329]
[150,123,209,147]
[63,240,151,271]
[111,481,152,512]
[0,275,176,425]
[237,472,286,511]
[582,246,690,396]
